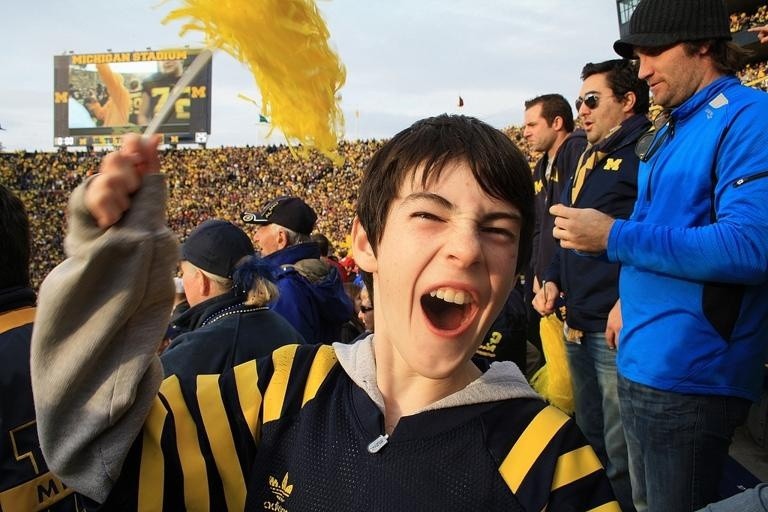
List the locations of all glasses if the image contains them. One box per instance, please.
[575,93,616,112]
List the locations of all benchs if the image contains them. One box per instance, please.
[711,362,768,502]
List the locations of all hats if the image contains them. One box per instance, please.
[613,0,733,60]
[178,219,255,280]
[240,196,318,234]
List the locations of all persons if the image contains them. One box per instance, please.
[1,116,625,347]
[543,0,767,512]
[70,59,191,126]
[0,183,87,511]
[26,111,625,511]
[157,216,310,378]
[530,61,649,510]
[721,1,767,94]
